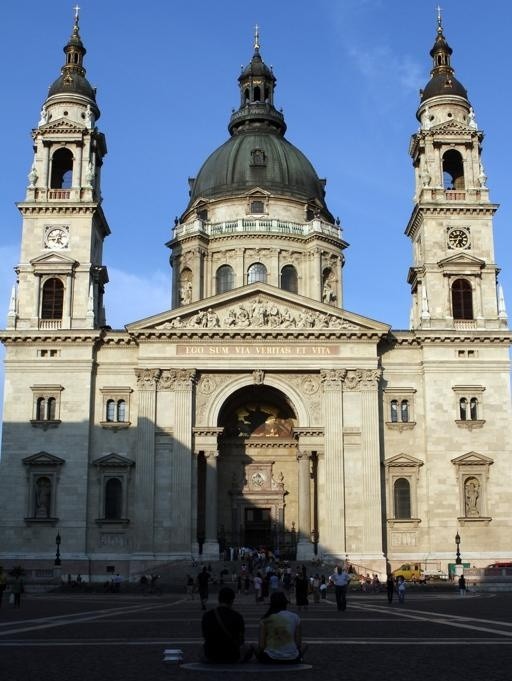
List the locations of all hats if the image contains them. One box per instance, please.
[269,591,292,604]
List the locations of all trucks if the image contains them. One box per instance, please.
[392,562,449,584]
[485,563,512,576]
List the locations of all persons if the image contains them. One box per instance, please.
[249,592,309,664]
[372,575,380,594]
[103,543,354,613]
[385,574,394,606]
[163,297,346,329]
[11,572,25,610]
[365,575,371,593]
[396,575,407,604]
[200,587,254,666]
[0,566,9,608]
[458,575,466,598]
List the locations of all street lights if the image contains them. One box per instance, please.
[454,531,462,563]
[55,528,62,566]
